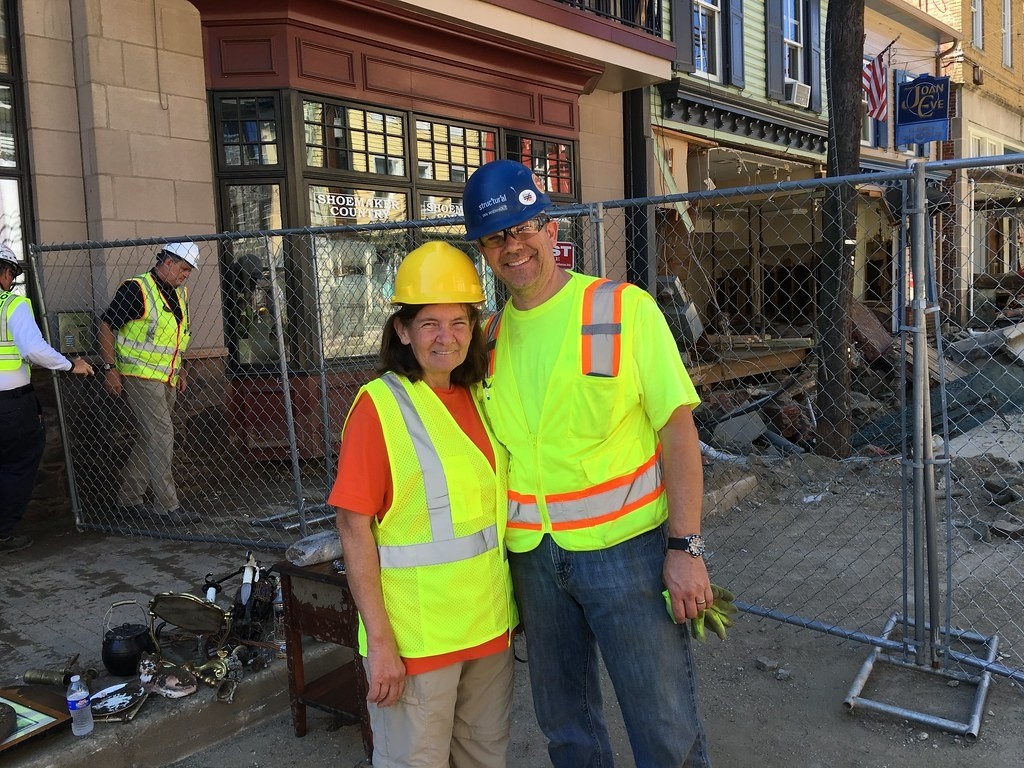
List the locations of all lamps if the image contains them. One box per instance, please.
[973,66,985,85]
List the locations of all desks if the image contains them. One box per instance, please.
[273,556,376,759]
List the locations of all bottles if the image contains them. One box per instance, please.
[273,582,286,650]
[66,675,94,737]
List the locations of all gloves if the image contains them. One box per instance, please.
[661,584,738,643]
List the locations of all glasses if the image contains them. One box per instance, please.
[477,213,550,248]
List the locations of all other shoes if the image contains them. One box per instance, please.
[153,507,203,525]
[0,534,34,555]
[119,503,154,519]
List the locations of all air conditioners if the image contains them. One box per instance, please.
[781,81,811,108]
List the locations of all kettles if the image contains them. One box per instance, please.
[101,598,167,678]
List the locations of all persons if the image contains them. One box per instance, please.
[98,242,201,525]
[463,161,713,768]
[234,254,285,364]
[328,240,523,768]
[0,243,94,552]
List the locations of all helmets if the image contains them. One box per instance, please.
[162,241,200,270]
[0,243,24,276]
[390,241,486,305]
[463,159,550,240]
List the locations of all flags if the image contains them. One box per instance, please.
[860,56,887,123]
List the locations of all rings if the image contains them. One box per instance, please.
[696,600,706,604]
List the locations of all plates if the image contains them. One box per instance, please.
[89,683,144,716]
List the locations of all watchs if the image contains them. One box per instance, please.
[103,363,115,371]
[668,534,706,558]
